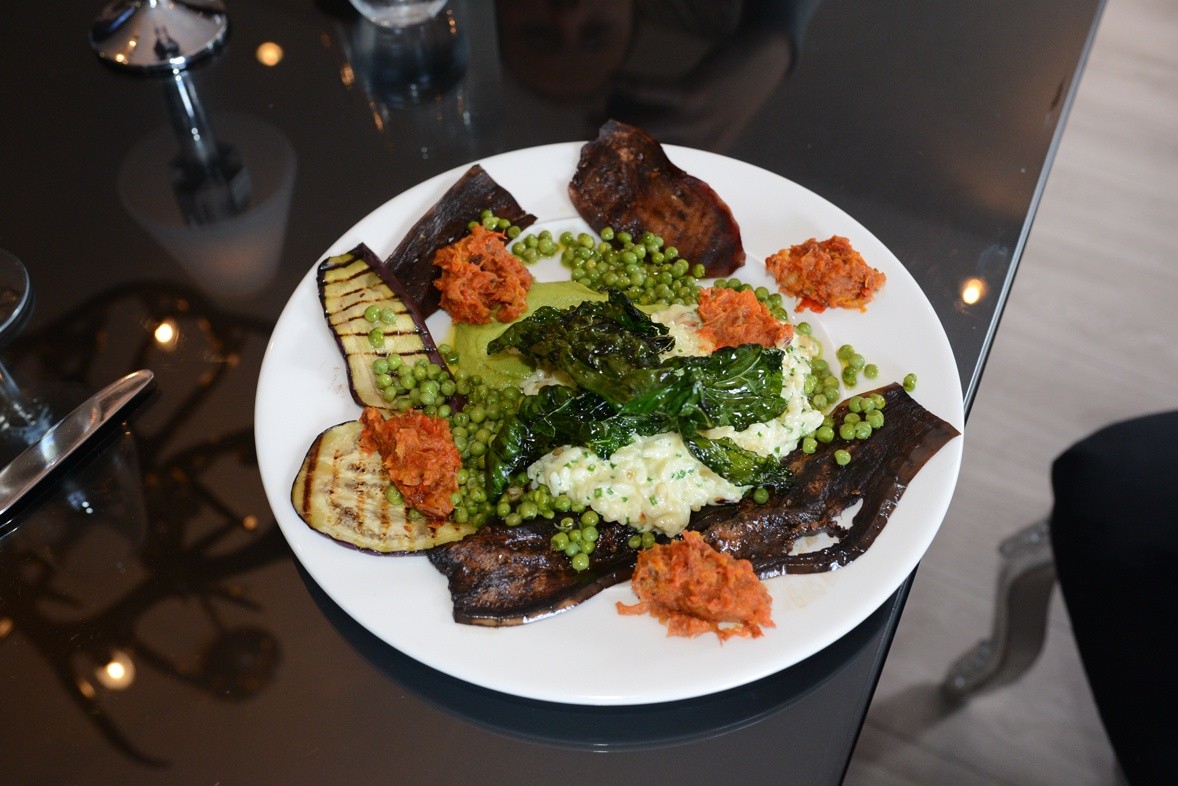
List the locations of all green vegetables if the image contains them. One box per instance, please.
[475,286,792,518]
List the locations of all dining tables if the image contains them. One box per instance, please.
[0,2,1111,786]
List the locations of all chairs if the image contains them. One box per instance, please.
[945,410,1178,786]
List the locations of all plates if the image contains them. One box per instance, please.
[253,138,969,713]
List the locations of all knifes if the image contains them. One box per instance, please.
[0,365,158,515]
